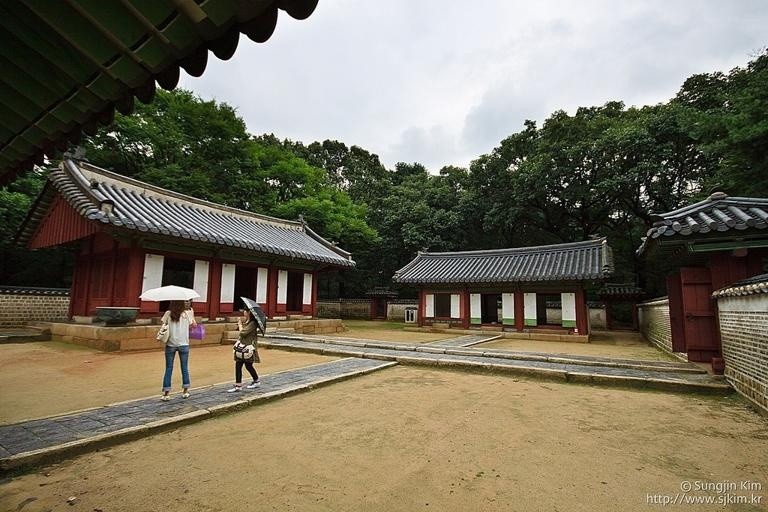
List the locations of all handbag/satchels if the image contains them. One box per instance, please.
[156,324,169,343]
[233,341,254,359]
[189,324,205,339]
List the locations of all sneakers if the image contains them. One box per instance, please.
[247,381,260,388]
[161,395,170,401]
[227,385,242,392]
[182,392,190,398]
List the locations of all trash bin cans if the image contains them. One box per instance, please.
[405,307,418,324]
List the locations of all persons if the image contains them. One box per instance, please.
[157,300,192,401]
[226,305,261,393]
[184,301,197,328]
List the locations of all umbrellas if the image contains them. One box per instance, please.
[237,296,266,337]
[138,284,201,303]
